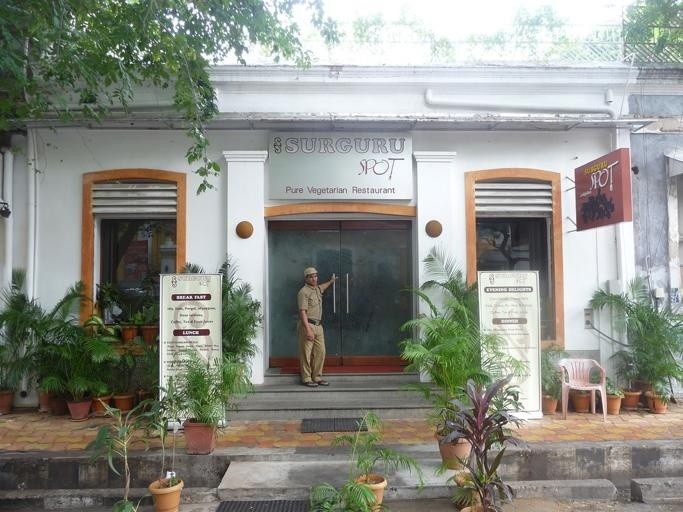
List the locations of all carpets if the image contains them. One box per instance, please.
[298,416,366,433]
[216,497,321,512]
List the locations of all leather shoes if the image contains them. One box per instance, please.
[316,380,329,386]
[305,381,318,387]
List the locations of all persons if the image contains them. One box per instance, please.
[295,267,340,388]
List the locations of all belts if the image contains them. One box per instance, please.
[307,318,321,325]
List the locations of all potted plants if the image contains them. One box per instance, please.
[310,413,426,511]
[2,269,160,421]
[125,376,187,511]
[541,274,683,414]
[160,351,254,453]
[396,246,531,511]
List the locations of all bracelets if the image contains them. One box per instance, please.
[328,281,331,285]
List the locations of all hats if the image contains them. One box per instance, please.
[304,267,318,278]
